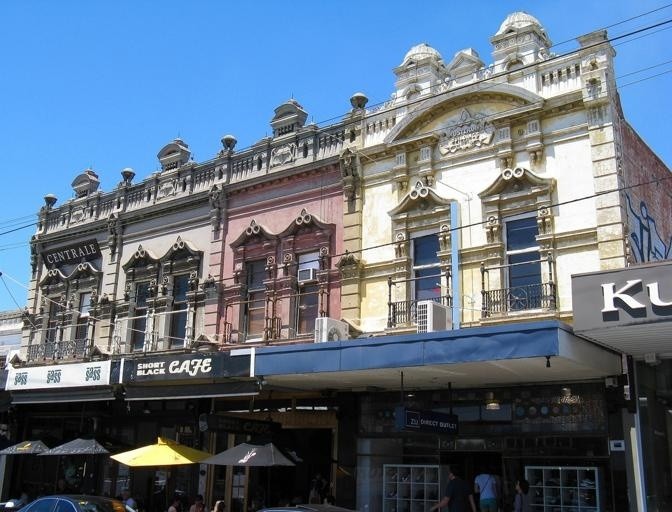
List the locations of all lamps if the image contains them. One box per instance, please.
[485,390,501,410]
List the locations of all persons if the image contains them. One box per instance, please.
[167,497,180,511]
[4,479,88,511]
[190,494,211,511]
[511,479,531,511]
[474,462,499,511]
[210,500,226,512]
[120,488,138,512]
[429,464,477,511]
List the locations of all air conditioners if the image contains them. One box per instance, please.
[298,268,319,282]
[416,299,453,333]
[314,317,350,343]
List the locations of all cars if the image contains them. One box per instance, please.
[17,494,135,512]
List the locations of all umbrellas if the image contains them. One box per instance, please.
[38,436,134,481]
[1,440,50,456]
[199,442,296,511]
[110,436,216,506]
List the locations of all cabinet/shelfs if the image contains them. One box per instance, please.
[524,464,612,512]
[382,463,451,512]
